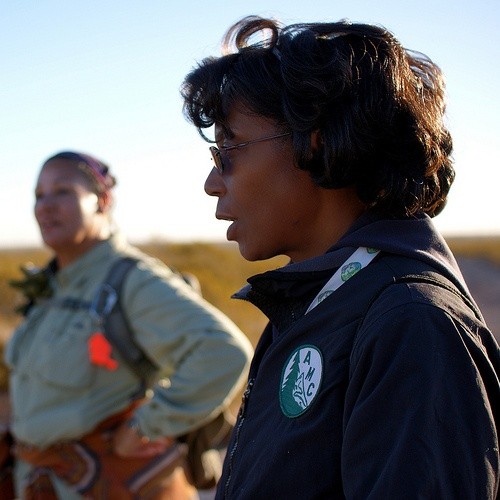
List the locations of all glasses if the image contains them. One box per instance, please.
[210,131,292,177]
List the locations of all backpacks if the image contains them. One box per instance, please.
[41,254,234,491]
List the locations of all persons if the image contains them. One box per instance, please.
[4,152,256,500]
[175,12,500,500]
